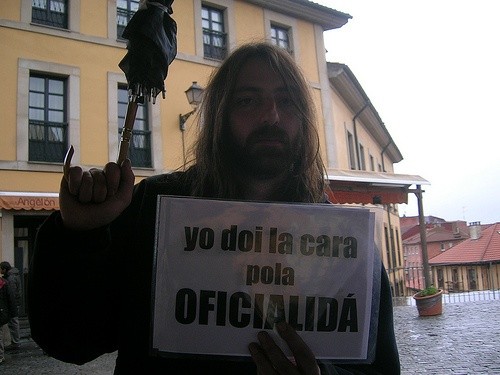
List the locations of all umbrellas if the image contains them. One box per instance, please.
[61,0,179,210]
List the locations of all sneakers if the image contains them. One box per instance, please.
[3,343,24,351]
[0,357,5,363]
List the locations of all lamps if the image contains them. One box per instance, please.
[179,82,206,132]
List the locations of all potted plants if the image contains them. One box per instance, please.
[415,284,444,316]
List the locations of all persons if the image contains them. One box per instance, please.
[0,261,22,363]
[25,41,401,375]
